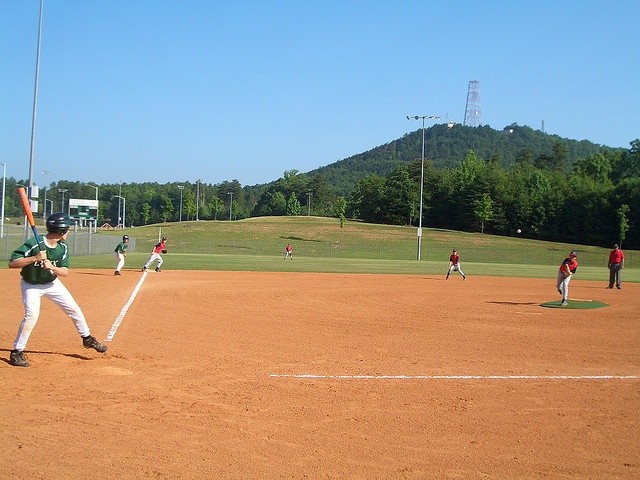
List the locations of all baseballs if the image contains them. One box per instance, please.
[516,229,521,234]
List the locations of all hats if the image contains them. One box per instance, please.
[570,252,577,257]
[614,244,619,248]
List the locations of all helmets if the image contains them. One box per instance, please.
[47,212,75,228]
[123,235,129,241]
[162,237,167,241]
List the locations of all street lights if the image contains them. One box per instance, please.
[84,184,99,224]
[115,195,126,230]
[406,115,441,260]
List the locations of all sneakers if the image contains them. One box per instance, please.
[142,266,146,271]
[607,286,613,289]
[10,350,29,367]
[155,267,161,272]
[616,286,621,289]
[115,271,121,275]
[557,289,562,294]
[81,335,108,353]
[560,299,568,306]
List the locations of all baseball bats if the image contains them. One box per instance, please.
[17,186,46,268]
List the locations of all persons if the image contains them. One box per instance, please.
[608,244,625,289]
[114,235,129,275]
[9,212,108,367]
[446,249,466,280]
[142,237,167,272]
[284,244,293,260]
[556,251,578,306]
